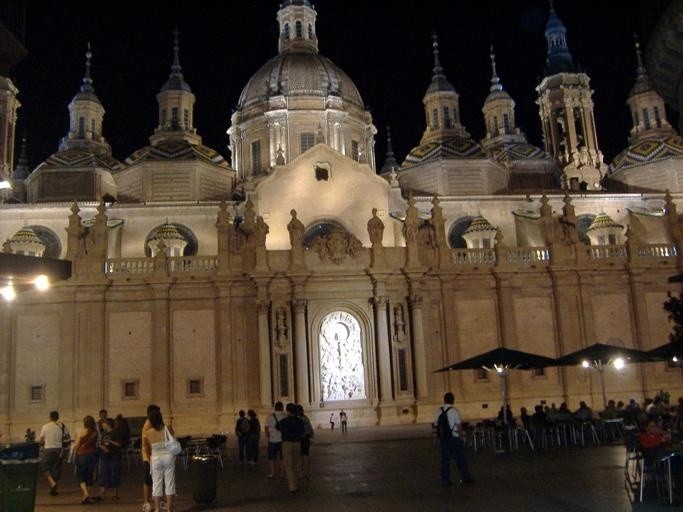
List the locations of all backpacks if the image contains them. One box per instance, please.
[437,407,456,442]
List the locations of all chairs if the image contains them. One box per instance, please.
[429,418,683,504]
[62,434,227,474]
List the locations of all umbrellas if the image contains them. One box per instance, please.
[433,347,557,424]
[558,343,670,409]
[651,344,683,359]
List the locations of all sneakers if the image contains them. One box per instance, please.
[160,499,167,510]
[142,503,152,512]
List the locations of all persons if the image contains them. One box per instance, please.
[265,401,289,478]
[25,409,131,505]
[145,409,176,512]
[340,409,347,433]
[235,410,250,463]
[330,413,334,429]
[296,404,314,479]
[142,405,160,512]
[498,390,682,464]
[434,392,474,487]
[247,409,260,464]
[274,403,306,493]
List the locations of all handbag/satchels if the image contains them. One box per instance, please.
[59,433,71,459]
[164,441,182,456]
